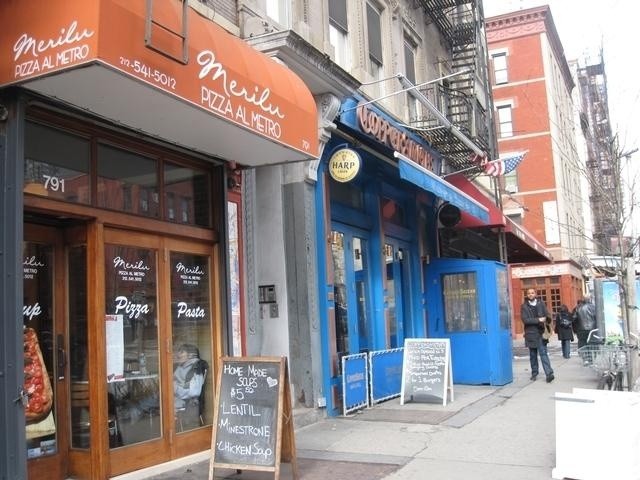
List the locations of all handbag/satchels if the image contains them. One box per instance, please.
[560,319,572,329]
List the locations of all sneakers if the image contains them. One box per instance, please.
[546,375,554,382]
[530,372,537,381]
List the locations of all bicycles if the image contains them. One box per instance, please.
[575,327,637,393]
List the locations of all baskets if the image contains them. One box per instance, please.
[577,345,629,371]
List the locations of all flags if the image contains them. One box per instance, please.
[477,151,525,178]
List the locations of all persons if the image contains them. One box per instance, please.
[572,300,582,356]
[572,296,596,366]
[554,304,574,359]
[519,288,555,383]
[116,344,206,427]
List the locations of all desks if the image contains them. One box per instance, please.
[70,373,159,385]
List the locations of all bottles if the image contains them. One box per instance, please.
[139,352,147,373]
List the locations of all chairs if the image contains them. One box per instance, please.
[69,380,119,449]
[149,359,208,432]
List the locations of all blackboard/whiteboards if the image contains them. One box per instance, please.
[402,338,456,402]
[210,355,296,472]
